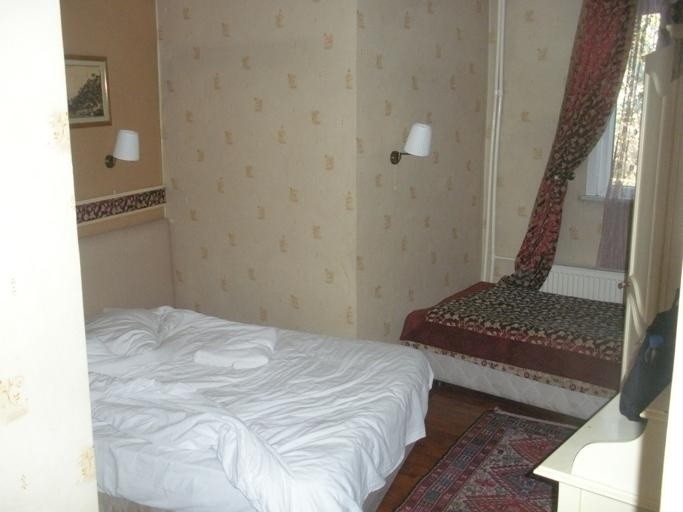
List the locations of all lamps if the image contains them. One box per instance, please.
[391,122,433,165]
[105,128,141,167]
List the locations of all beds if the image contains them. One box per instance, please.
[82,302,434,511]
[400,281,621,423]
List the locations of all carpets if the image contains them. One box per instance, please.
[392,406,579,512]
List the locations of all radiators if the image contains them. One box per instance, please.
[535,263,624,303]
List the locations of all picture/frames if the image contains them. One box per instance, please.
[65,54,111,129]
